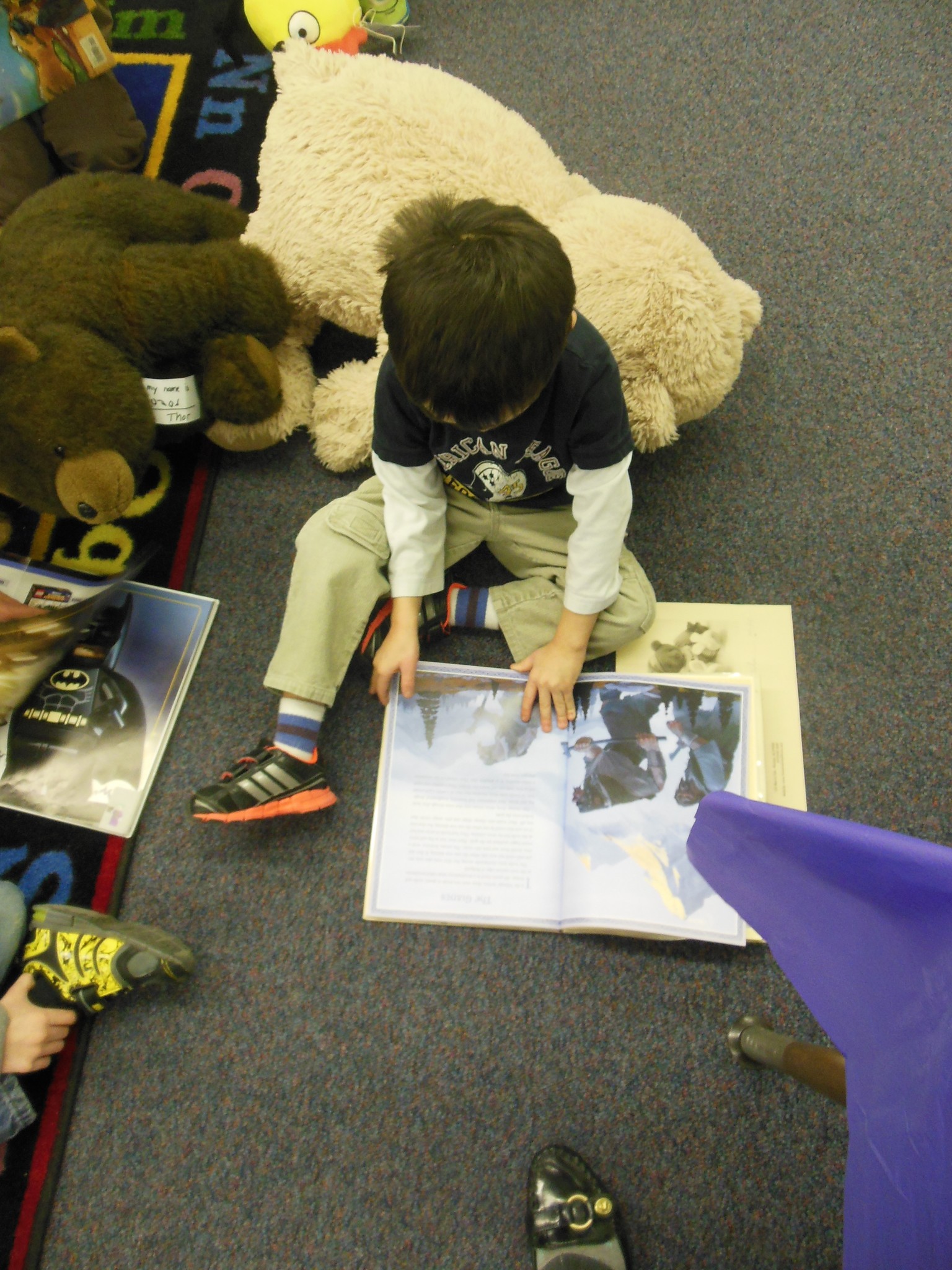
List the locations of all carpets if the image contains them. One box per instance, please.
[1,1,278,1270]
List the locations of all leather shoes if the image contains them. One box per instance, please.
[524,1145,634,1270]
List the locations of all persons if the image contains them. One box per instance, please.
[0,594,196,1150]
[471,682,744,816]
[526,1145,629,1270]
[188,200,658,824]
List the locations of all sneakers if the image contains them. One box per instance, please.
[24,905,195,1014]
[190,738,335,821]
[361,583,465,659]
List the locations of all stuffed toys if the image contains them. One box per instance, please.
[0,3,763,528]
